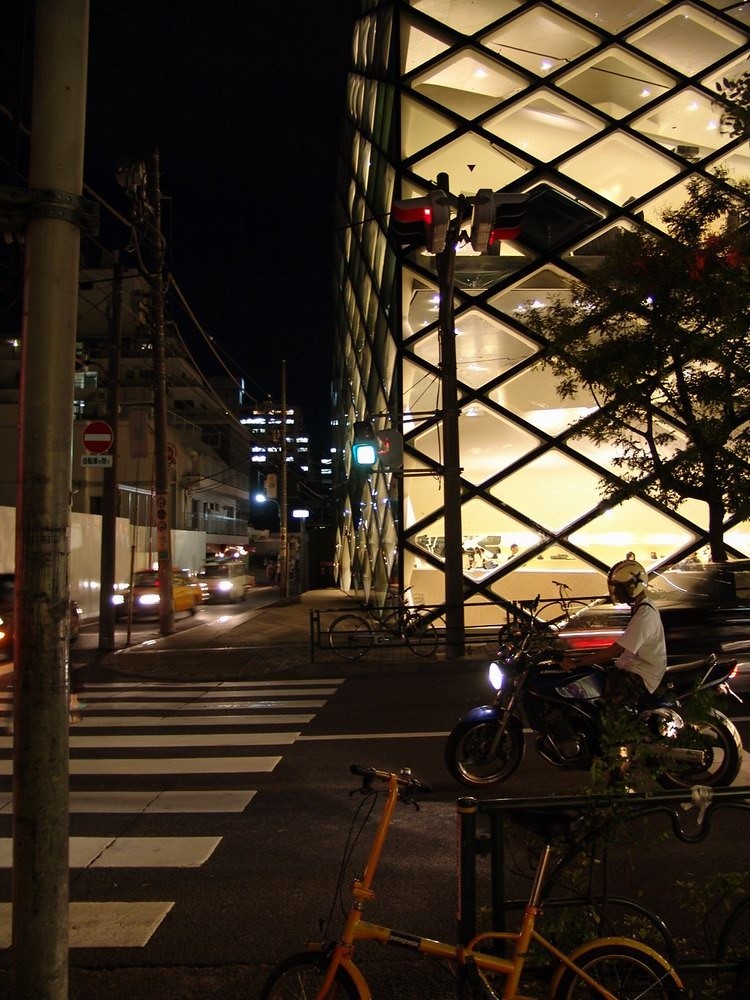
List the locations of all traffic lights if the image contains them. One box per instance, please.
[351,420,379,466]
[378,428,403,472]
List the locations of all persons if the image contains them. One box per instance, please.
[266,558,299,587]
[575,562,667,789]
[416,535,713,571]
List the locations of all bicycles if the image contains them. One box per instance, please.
[498,581,572,648]
[255,761,687,1000]
[327,585,439,660]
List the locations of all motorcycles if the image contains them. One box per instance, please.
[442,597,744,790]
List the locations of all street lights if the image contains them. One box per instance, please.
[293,509,309,593]
[256,494,281,524]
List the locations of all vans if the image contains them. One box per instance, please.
[191,556,249,606]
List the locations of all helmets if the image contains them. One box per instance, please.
[607,561,648,606]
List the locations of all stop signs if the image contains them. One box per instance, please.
[82,422,114,453]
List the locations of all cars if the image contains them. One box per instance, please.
[114,563,204,621]
[0,573,81,653]
